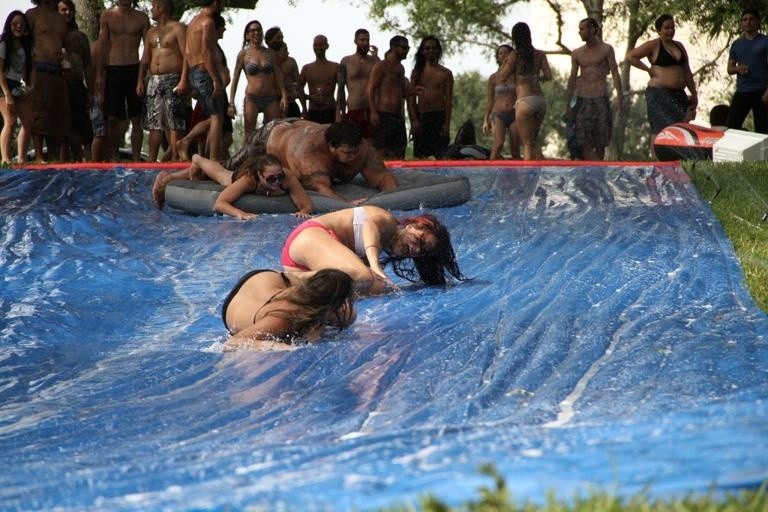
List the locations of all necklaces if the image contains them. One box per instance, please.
[258,185,276,197]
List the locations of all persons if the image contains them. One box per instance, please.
[298,29,380,139]
[367,35,425,164]
[483,44,523,160]
[494,22,551,161]
[97,2,232,164]
[190,152,314,219]
[266,27,301,117]
[220,268,357,352]
[566,17,624,160]
[152,116,398,210]
[406,36,452,162]
[710,105,748,131]
[227,21,288,142]
[626,14,699,160]
[726,8,768,134]
[1,1,107,163]
[281,205,466,296]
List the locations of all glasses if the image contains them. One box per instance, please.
[262,170,286,183]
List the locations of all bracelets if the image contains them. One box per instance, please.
[269,341,277,350]
